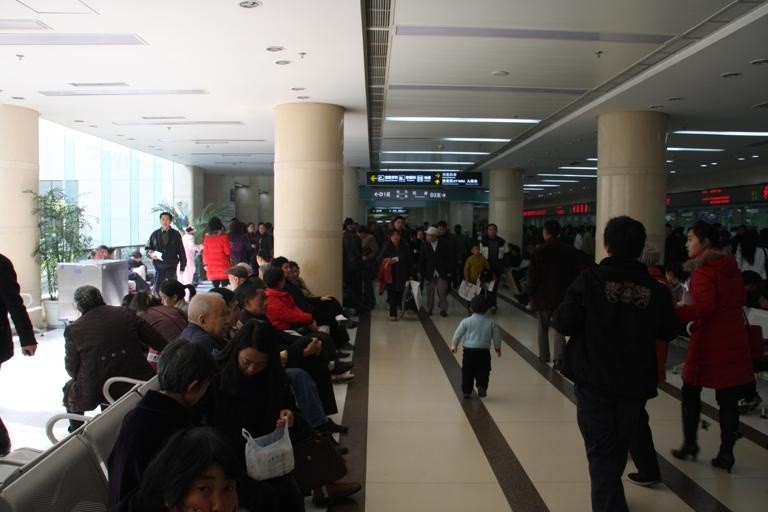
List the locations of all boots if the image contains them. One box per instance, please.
[671,401,702,461]
[711,409,739,474]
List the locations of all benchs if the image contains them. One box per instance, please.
[667,306,768,419]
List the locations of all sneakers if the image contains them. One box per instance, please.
[628,472,662,486]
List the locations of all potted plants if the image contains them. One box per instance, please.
[22,188,93,328]
[151,202,233,288]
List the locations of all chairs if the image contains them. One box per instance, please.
[0,323,330,512]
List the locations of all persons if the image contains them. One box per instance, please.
[89,211,274,298]
[664,223,691,304]
[343,216,546,323]
[0,254,38,457]
[556,224,596,258]
[714,223,768,372]
[671,220,755,474]
[553,216,679,512]
[451,296,501,398]
[60,249,360,512]
[527,219,582,373]
[627,241,673,484]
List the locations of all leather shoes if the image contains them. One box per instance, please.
[311,316,361,507]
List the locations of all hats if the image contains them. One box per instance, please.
[225,266,247,279]
[424,227,439,236]
